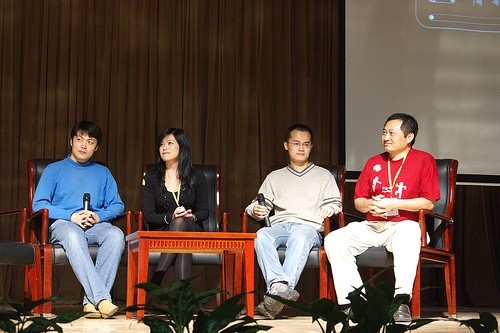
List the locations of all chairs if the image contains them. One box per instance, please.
[242,166,347,313]
[0,158,132,319]
[329,158,460,319]
[134,164,230,233]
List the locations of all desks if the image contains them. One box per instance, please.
[126,231,257,323]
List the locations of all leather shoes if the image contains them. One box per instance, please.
[393,303,414,322]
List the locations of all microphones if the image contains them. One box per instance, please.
[83,192,91,227]
[257,193,271,227]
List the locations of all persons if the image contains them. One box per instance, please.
[324,114,440,321]
[245,122,342,318]
[33,121,125,319]
[141,127,209,309]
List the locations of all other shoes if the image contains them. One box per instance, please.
[263,282,301,312]
[98,300,119,319]
[256,302,281,320]
[82,303,102,320]
